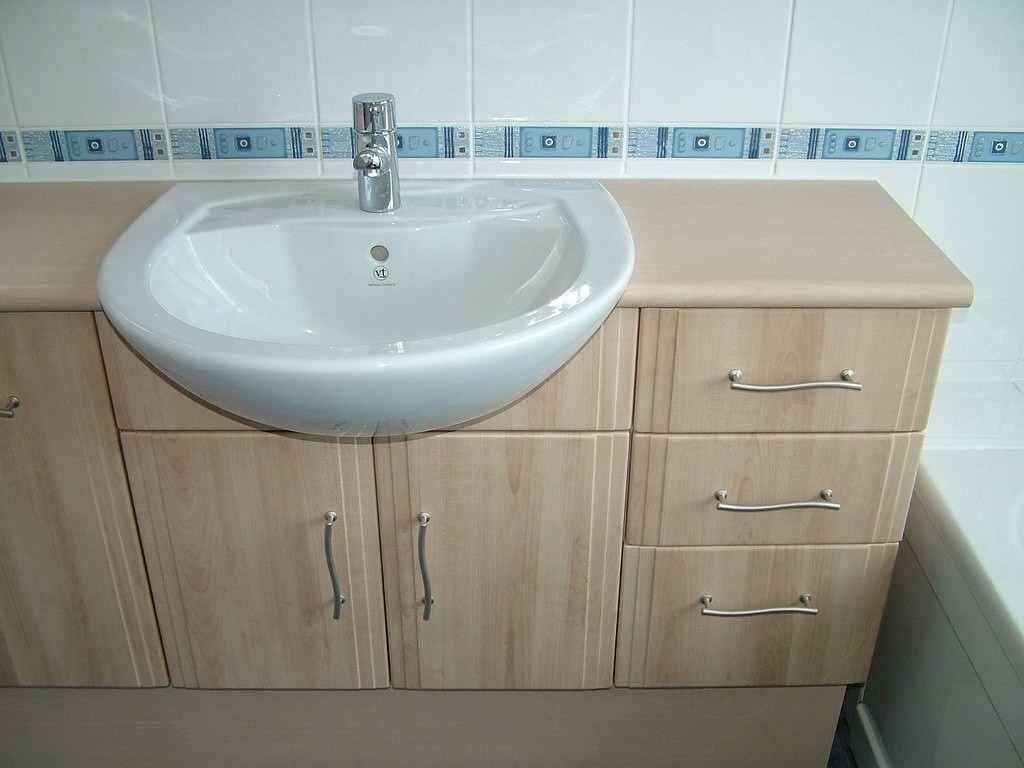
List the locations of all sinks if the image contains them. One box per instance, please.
[95,177,636,439]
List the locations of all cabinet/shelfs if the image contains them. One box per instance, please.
[1,303,951,691]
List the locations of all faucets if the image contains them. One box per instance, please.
[351,92,402,213]
